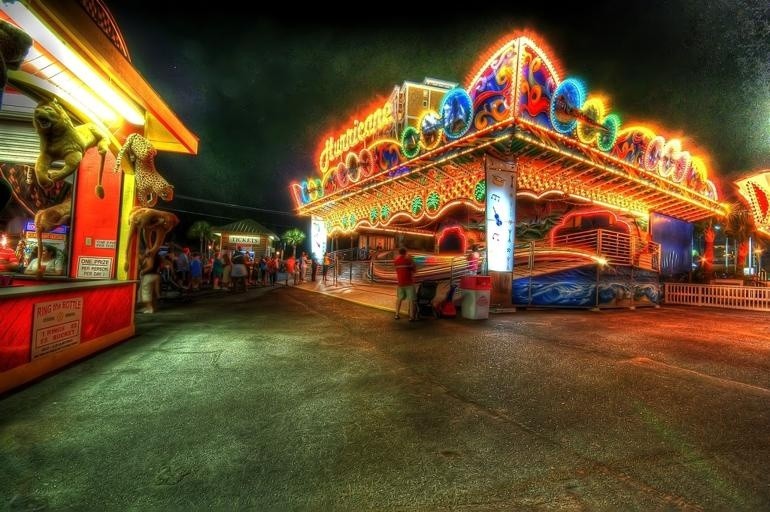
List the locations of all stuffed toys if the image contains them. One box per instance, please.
[32,198,73,281]
[113,132,176,209]
[32,97,112,200]
[122,208,179,273]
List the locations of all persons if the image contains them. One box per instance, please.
[138,245,335,295]
[393,247,418,322]
[466,245,481,275]
[15,240,30,273]
[136,245,166,315]
[24,244,64,277]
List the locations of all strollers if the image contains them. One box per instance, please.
[410,280,440,319]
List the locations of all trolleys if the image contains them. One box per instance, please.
[436,284,458,319]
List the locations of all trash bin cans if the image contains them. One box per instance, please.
[460,276,494,320]
